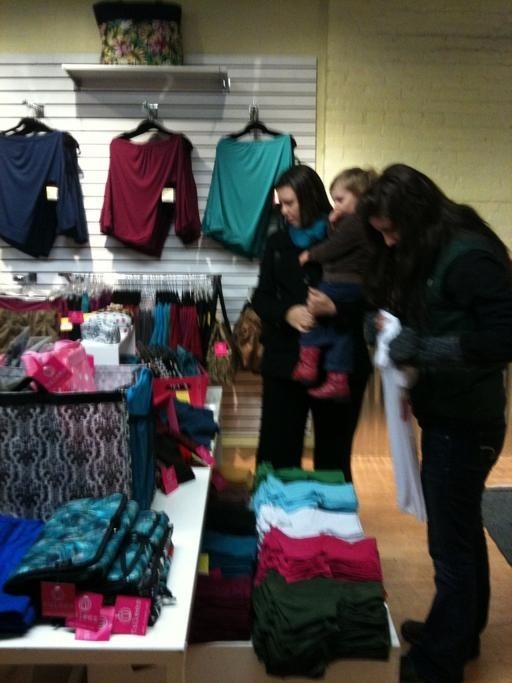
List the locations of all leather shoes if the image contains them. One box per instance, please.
[400,620,480,683]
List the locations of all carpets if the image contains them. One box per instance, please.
[479,485,511,563]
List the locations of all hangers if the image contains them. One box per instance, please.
[2,268,211,315]
[114,100,177,143]
[1,98,80,148]
[227,104,300,148]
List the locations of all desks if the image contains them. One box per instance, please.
[1,382,225,682]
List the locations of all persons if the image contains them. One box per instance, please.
[356,164,512,683]
[249,166,373,507]
[291,166,383,400]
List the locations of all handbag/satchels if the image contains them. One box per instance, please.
[207,299,265,385]
[92,3,183,65]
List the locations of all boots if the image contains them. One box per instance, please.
[308,372,350,399]
[291,346,320,380]
